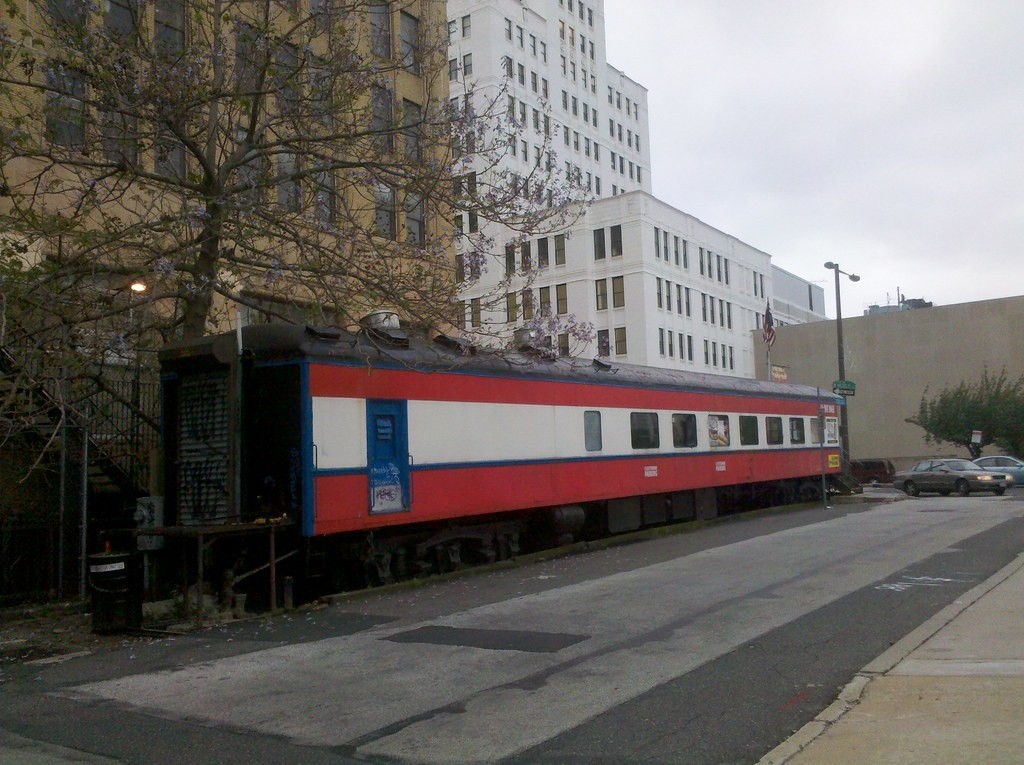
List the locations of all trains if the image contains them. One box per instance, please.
[147,321,844,614]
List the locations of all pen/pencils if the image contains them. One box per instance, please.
[87,551,143,635]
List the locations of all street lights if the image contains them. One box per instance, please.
[824,261,860,489]
[128,281,147,326]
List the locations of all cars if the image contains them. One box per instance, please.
[971,456,1024,485]
[894,459,1014,497]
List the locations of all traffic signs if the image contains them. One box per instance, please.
[832,387,856,397]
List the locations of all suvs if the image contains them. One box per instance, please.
[850,458,896,484]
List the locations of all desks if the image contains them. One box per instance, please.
[102,520,298,629]
